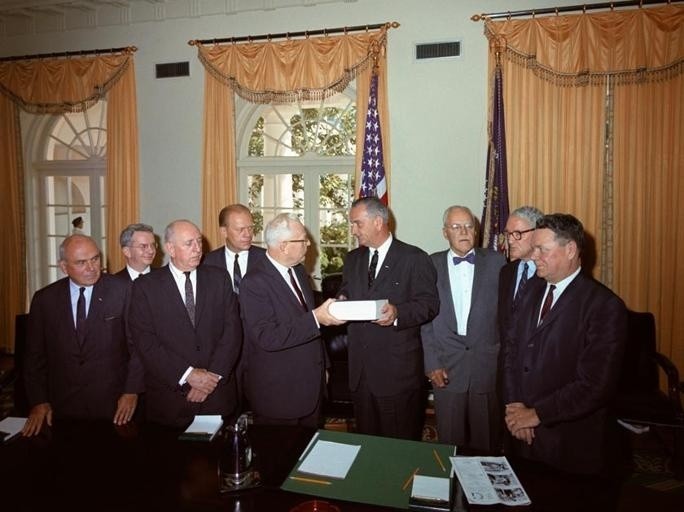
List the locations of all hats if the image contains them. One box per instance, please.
[71,216,83,227]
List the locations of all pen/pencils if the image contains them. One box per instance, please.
[209,422,224,442]
[402,467,420,491]
[4,426,23,440]
[433,448,446,472]
[289,476,331,486]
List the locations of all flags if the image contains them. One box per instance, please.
[358,55,388,206]
[478,65,510,250]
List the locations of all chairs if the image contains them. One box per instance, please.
[619,312,680,428]
[320,272,351,420]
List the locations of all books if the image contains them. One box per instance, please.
[408,473,451,511]
[178,415,223,442]
[0,417,28,442]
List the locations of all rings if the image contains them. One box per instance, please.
[431,375,436,380]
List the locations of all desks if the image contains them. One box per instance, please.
[0,416,684,512]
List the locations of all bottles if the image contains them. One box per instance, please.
[218,426,263,494]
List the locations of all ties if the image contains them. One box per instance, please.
[287,268,309,313]
[183,272,196,331]
[367,248,382,287]
[513,262,530,312]
[233,253,242,298]
[537,284,557,333]
[76,287,89,346]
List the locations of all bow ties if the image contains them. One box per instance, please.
[451,252,477,265]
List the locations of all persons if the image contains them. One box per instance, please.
[200,204,268,410]
[22,236,145,439]
[128,219,244,423]
[498,207,544,415]
[336,196,441,442]
[239,213,347,430]
[503,212,628,465]
[113,224,157,281]
[71,217,87,236]
[421,206,507,453]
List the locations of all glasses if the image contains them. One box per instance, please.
[502,226,539,241]
[443,222,475,231]
